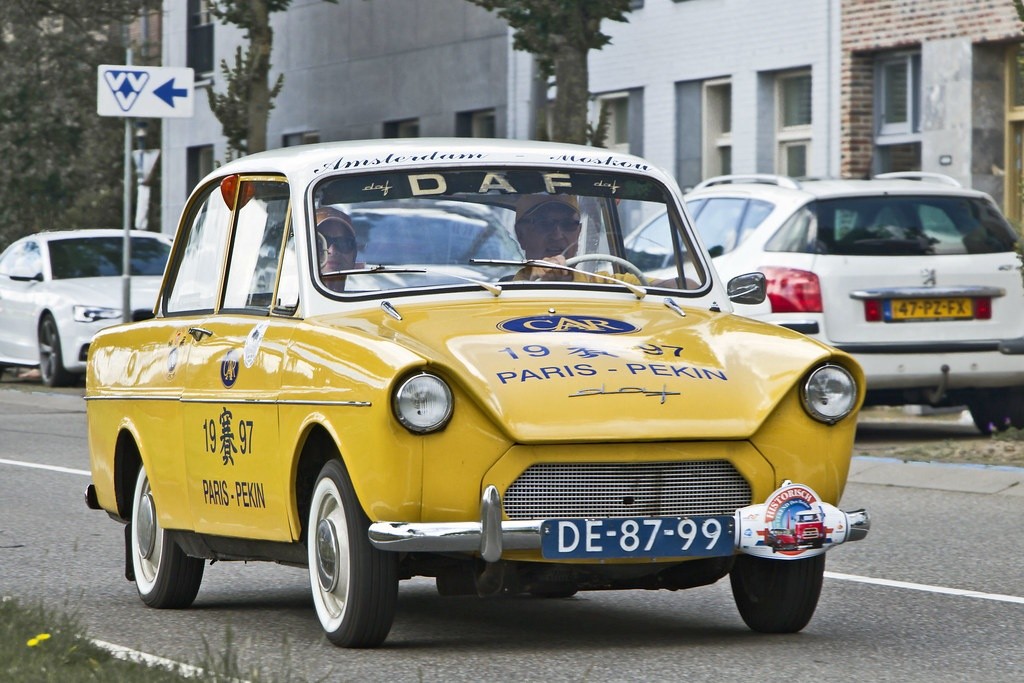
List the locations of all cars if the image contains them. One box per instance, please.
[0,229,173,387]
[82,136,869,650]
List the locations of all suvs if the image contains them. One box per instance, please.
[626,171,1023,435]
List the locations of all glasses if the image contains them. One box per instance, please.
[324,236,357,254]
[520,219,579,231]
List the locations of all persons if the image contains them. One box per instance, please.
[312,207,358,278]
[488,192,701,289]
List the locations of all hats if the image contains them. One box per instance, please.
[516,194,580,222]
[315,207,356,238]
